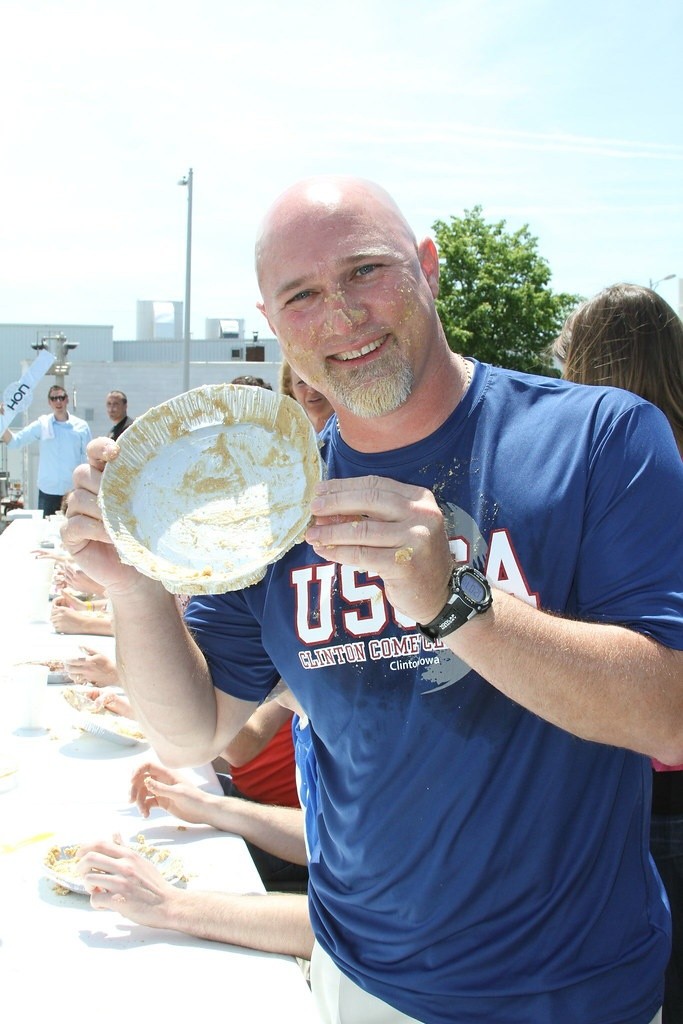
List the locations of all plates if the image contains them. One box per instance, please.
[14,661,72,682]
[45,833,188,894]
[98,384,322,593]
[59,685,146,747]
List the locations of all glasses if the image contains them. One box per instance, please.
[245,376,253,385]
[49,395,67,401]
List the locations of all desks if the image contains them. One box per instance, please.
[0,519,313,1024]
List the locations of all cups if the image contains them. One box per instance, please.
[13,665,49,730]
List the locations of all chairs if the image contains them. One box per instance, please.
[1,496,25,516]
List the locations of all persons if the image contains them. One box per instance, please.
[59,169,682,1023]
[560,284,682,1024]
[0,386,93,522]
[61,362,336,966]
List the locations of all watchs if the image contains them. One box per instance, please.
[414,565,494,644]
